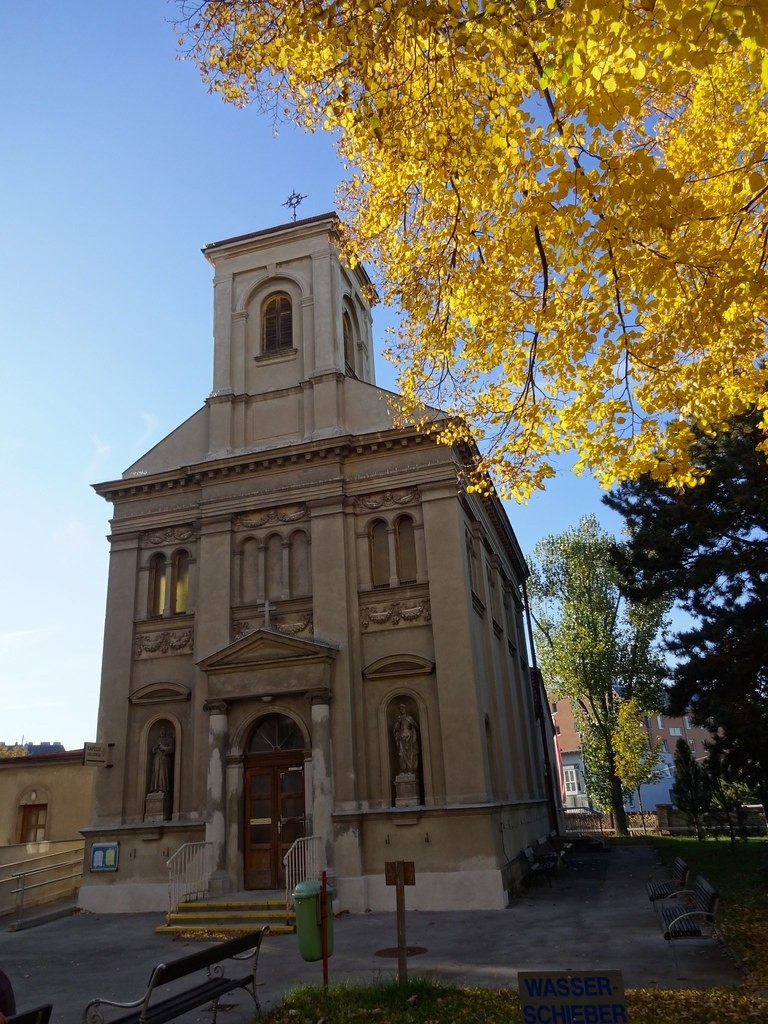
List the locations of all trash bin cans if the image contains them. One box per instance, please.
[291,880,335,963]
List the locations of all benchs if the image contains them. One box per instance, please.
[82,926,271,1024]
[549,829,573,855]
[535,835,570,868]
[520,845,557,887]
[657,875,743,980]
[4,1003,54,1024]
[646,856,690,913]
